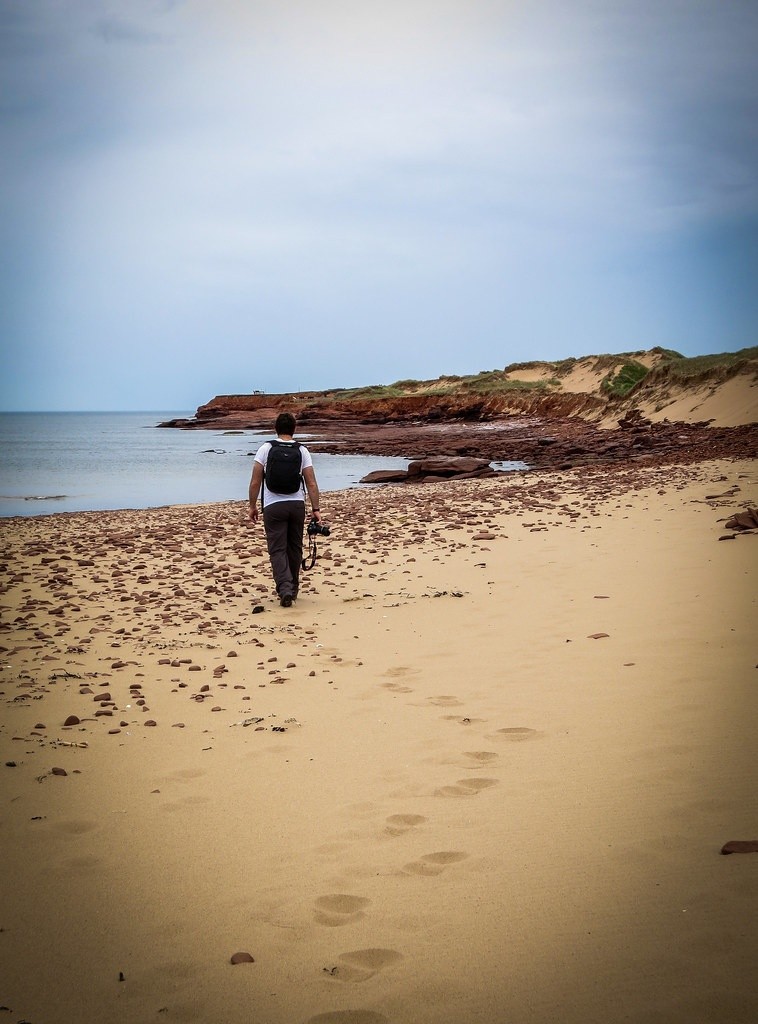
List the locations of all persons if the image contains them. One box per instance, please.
[248,413,322,604]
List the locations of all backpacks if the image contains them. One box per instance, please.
[260,440,306,512]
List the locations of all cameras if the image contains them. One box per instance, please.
[307,518,330,536]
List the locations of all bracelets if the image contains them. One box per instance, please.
[312,508,320,512]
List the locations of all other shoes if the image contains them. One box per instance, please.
[292,592,298,601]
[280,592,292,607]
